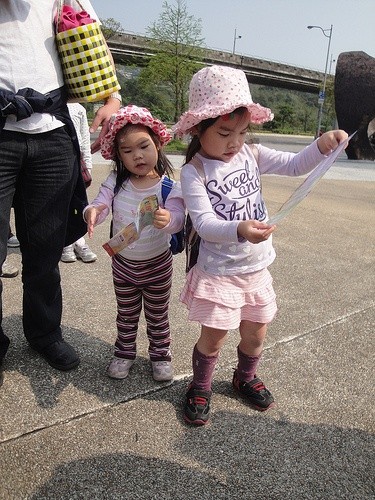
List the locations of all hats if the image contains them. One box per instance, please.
[171,64,274,135]
[100,105,172,160]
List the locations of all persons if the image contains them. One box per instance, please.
[171,65,348,425]
[0,0,123,371]
[83,105,185,381]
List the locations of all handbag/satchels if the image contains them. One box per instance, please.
[56,0,121,105]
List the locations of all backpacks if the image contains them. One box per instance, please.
[109,172,186,254]
[183,155,208,273]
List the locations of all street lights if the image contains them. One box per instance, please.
[329,55,336,73]
[307,25,333,140]
[232,28,242,54]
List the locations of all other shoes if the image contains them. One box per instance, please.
[1,261,19,278]
[7,235,20,247]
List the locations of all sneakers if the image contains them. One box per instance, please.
[232,368,275,410]
[60,246,76,262]
[0,335,9,365]
[107,357,135,379]
[74,242,97,262]
[183,380,213,425]
[27,332,80,370]
[152,361,173,381]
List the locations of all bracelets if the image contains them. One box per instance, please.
[104,92,122,105]
